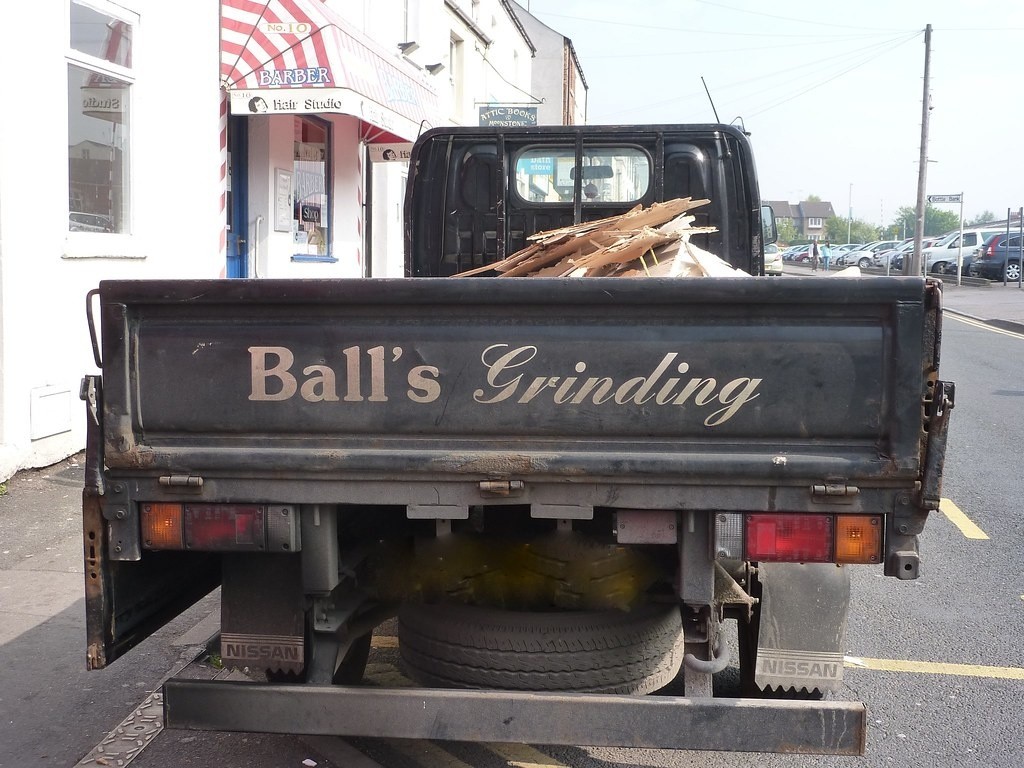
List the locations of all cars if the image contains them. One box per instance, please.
[763,243,783,276]
[782,227,1024,282]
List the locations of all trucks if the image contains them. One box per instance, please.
[79,123,956,755]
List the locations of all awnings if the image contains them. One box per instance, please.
[80,19,131,124]
[221,0,450,143]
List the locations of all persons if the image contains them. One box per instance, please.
[808,239,821,271]
[822,241,832,272]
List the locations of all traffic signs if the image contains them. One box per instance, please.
[926,194,961,203]
